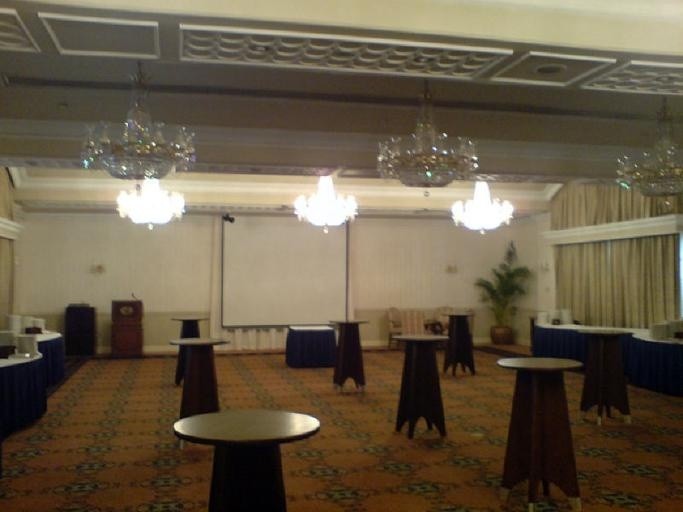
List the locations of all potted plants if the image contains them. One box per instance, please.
[473,241,529,345]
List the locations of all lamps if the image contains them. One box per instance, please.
[376,76,479,189]
[614,95,683,197]
[293,174,358,235]
[79,58,196,180]
[449,181,514,235]
[116,177,186,231]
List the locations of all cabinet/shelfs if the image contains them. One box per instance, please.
[111,300,143,357]
[64,303,97,357]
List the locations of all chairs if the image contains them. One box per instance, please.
[388,307,405,350]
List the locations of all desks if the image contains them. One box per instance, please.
[0,332,66,381]
[168,338,229,418]
[391,333,450,440]
[285,325,337,369]
[0,350,48,441]
[496,357,584,512]
[329,320,370,393]
[173,410,321,512]
[172,314,209,339]
[577,328,636,426]
[629,329,683,397]
[534,321,601,373]
[441,312,477,378]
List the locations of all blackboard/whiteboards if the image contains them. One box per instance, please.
[220,214,349,327]
